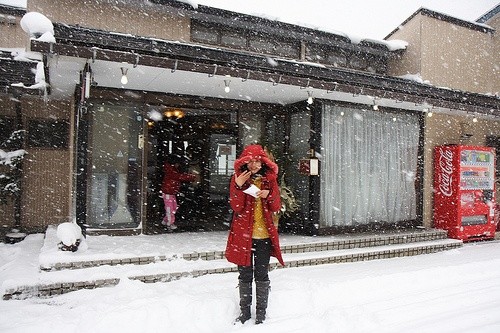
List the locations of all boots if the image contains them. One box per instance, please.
[254,281,271,324]
[235,278,253,323]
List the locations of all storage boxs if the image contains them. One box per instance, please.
[298,159,318,175]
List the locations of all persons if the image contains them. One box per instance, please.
[162,157,196,229]
[225,145,286,326]
